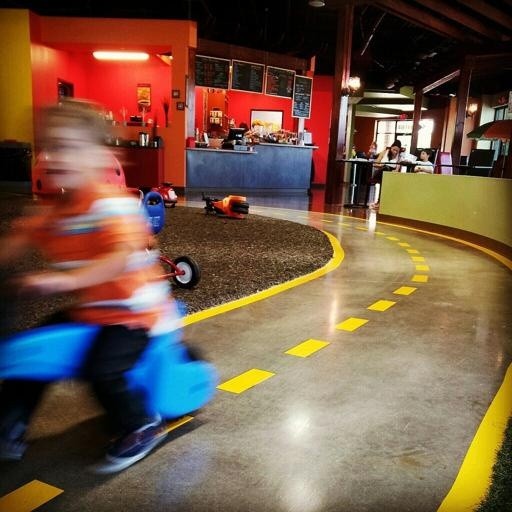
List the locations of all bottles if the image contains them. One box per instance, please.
[139,131,146,147]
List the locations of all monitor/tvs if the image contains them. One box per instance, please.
[228,128,245,140]
[250,109,283,132]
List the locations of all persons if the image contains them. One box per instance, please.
[367,139,401,209]
[0,95,189,476]
[411,149,434,175]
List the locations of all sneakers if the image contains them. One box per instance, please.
[104,409,168,468]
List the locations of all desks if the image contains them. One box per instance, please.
[335,158,376,209]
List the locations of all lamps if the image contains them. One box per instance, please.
[343,70,361,97]
[466,103,478,119]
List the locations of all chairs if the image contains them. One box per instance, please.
[365,146,495,210]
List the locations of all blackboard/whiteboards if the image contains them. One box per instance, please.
[230,59,266,94]
[194,55,231,91]
[264,66,297,99]
[291,75,313,119]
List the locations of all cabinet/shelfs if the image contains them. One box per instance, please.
[105,146,165,191]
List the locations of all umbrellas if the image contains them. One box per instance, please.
[464,119,512,178]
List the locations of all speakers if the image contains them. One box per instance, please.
[460,156,467,165]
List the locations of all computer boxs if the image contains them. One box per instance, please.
[468,149,495,166]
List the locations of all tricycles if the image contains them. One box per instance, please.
[144,232,203,293]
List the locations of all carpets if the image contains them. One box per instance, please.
[478,408,512,508]
[0,193,334,332]
